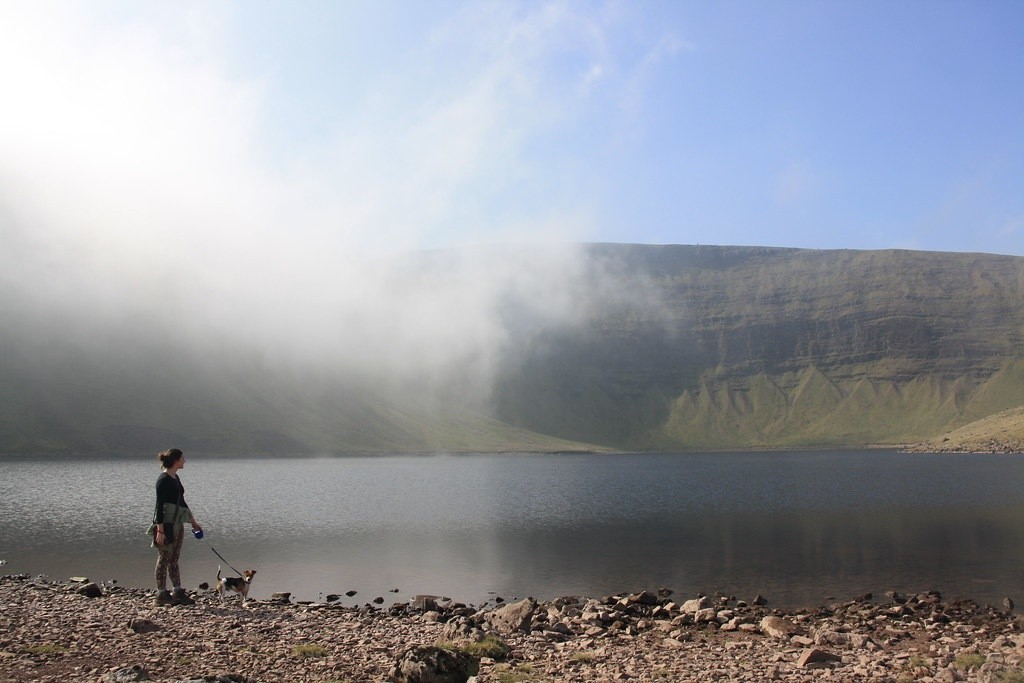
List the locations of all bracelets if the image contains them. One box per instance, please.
[157,529,165,534]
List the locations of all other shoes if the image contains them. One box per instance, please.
[171,588,195,605]
[153,589,179,607]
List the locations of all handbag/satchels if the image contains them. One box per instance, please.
[154,522,175,545]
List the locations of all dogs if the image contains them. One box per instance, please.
[216,564,257,605]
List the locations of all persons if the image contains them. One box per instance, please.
[145,448,203,605]
